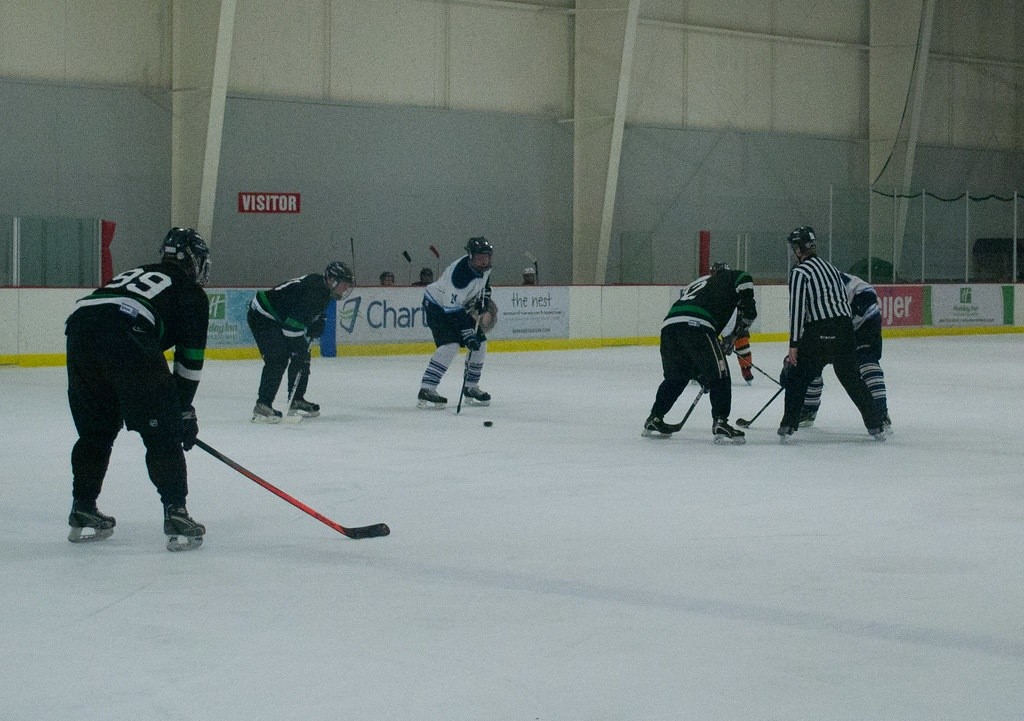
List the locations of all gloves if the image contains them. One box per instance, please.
[475,295,493,314]
[307,313,326,338]
[179,402,199,451]
[292,347,311,373]
[463,325,480,351]
[721,334,734,357]
[737,316,756,336]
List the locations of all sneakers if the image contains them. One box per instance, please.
[464,385,492,406]
[250,399,283,425]
[163,498,206,551]
[712,415,746,445]
[417,388,448,410]
[797,408,818,427]
[777,422,798,444]
[67,499,116,543]
[882,407,893,436]
[641,415,673,440]
[741,365,754,384]
[865,424,886,442]
[287,395,321,418]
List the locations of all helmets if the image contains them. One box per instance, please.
[521,268,535,276]
[788,225,815,254]
[325,261,355,298]
[161,225,211,286]
[465,235,493,274]
[710,261,731,274]
[379,272,395,284]
[419,268,433,280]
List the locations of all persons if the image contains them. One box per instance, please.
[380,271,395,286]
[799,272,891,428]
[777,226,884,436]
[418,235,492,403]
[411,268,433,286]
[520,267,534,285]
[63,226,212,536]
[247,261,355,418]
[643,261,757,438]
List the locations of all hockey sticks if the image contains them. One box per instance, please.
[736,387,784,429]
[730,347,782,386]
[280,338,314,424]
[445,314,482,415]
[192,438,391,539]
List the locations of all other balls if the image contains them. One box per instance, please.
[484,421,491,427]
